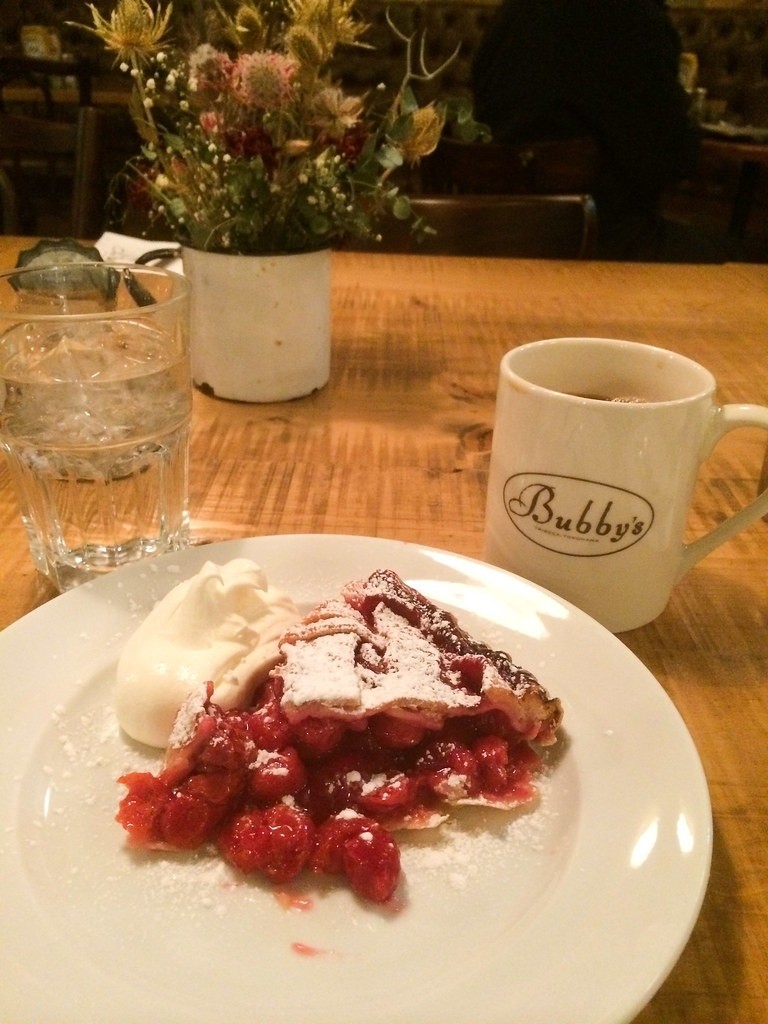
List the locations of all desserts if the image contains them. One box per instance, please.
[108,555,563,905]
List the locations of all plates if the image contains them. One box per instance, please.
[0,532,713,1024]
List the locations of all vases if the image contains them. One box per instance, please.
[177,245,334,404]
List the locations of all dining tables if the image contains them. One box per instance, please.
[1,234,768,1024]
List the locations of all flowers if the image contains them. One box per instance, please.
[59,0,497,257]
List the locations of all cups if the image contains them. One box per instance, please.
[480,332,766,632]
[1,260,198,599]
[132,240,342,407]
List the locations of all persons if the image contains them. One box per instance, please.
[468,0,704,247]
